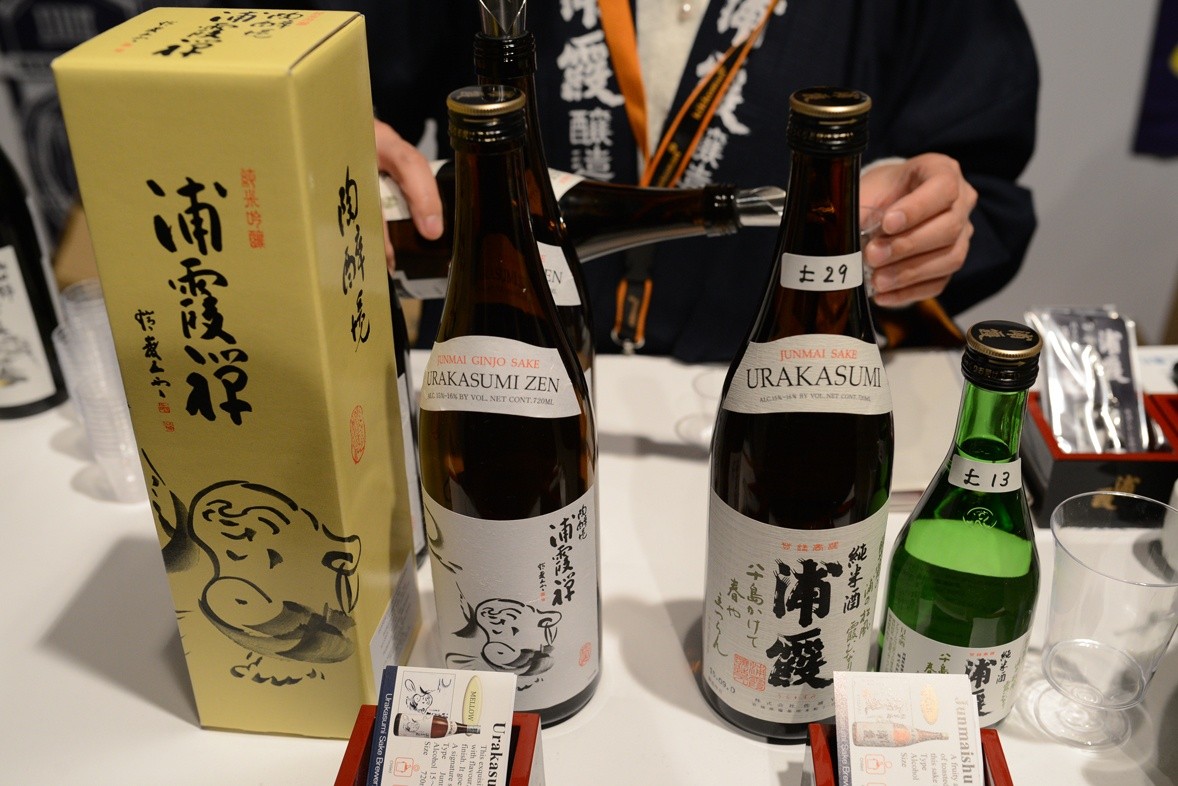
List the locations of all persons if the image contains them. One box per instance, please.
[323,0,1040,367]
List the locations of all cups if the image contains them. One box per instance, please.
[53,279,151,503]
[1036,489,1178,746]
[1161,479,1178,572]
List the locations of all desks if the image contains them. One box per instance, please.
[1,283,1178,784]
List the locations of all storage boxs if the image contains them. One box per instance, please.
[51,6,429,748]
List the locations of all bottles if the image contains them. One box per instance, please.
[416,0,603,730]
[379,156,741,300]
[702,89,895,736]
[1,145,70,419]
[874,322,1041,729]
